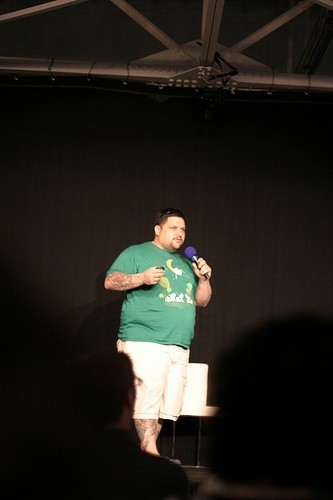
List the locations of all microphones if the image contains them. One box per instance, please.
[184,246,209,279]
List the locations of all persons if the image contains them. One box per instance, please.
[74,351,191,500]
[0,328,73,500]
[190,308,333,499]
[104,208,213,465]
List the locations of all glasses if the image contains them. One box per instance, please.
[159,208,183,218]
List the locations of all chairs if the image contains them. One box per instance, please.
[159,362,220,469]
